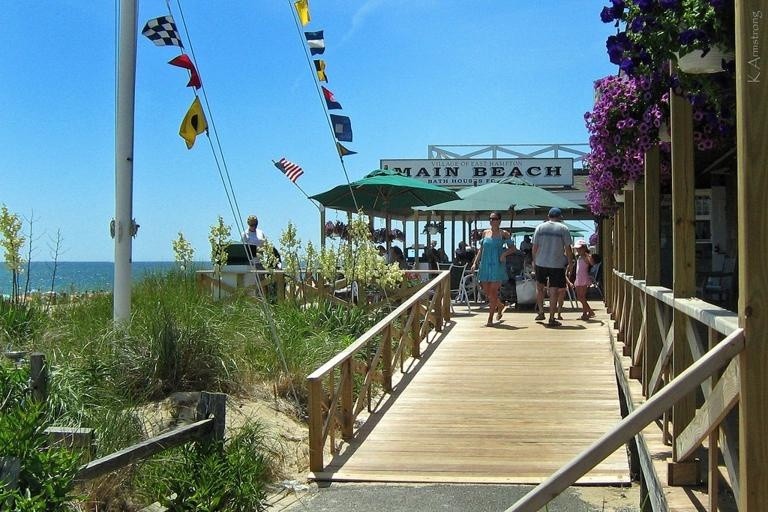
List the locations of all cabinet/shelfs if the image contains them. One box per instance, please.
[664,187,726,273]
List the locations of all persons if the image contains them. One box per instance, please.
[531,207,575,327]
[239,214,267,281]
[470,211,515,327]
[365,237,600,327]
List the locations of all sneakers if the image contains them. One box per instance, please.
[535,313,563,326]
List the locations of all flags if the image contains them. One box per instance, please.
[273,157,304,183]
[314,59,329,83]
[141,15,184,48]
[329,114,353,142]
[167,53,201,91]
[294,1,311,27]
[303,30,326,56]
[321,86,343,111]
[177,95,210,150]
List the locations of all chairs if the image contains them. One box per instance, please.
[323,259,603,312]
[696,245,738,309]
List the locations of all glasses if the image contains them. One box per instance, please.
[249,224,257,227]
[488,218,500,221]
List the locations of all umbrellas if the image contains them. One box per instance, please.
[469,220,585,247]
[306,168,463,258]
[413,175,585,247]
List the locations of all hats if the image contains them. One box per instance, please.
[548,207,563,218]
[572,240,586,248]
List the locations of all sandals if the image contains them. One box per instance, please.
[487,303,505,327]
[576,313,595,320]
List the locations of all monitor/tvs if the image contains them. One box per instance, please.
[405,248,425,262]
